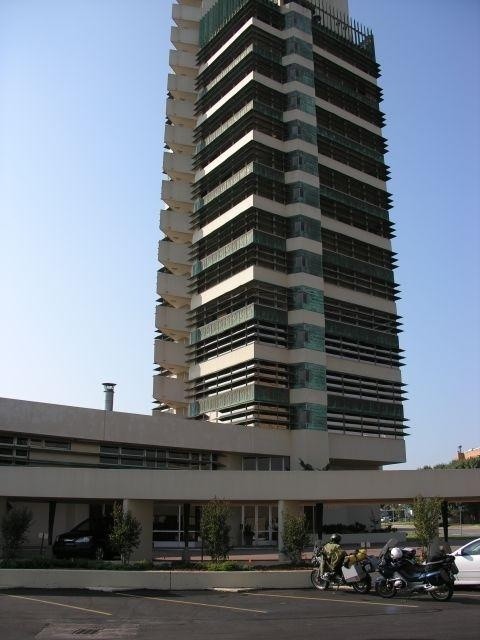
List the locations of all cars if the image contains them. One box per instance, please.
[179,529,213,543]
[380,509,398,522]
[420,538,480,593]
[264,514,279,540]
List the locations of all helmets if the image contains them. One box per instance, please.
[330,535,340,543]
[391,548,402,560]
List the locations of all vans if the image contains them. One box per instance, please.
[51,515,154,561]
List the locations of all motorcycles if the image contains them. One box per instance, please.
[310,534,376,595]
[375,532,459,602]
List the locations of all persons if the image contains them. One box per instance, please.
[322,533,347,571]
[429,540,451,561]
